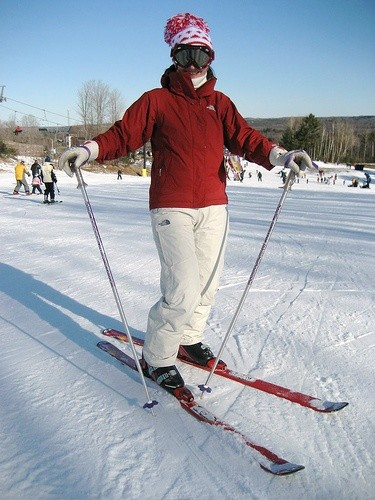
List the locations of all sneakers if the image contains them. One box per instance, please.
[178,342,215,366]
[143,354,185,388]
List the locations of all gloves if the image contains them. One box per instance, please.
[269,145,313,177]
[57,139,100,177]
[54,179,57,183]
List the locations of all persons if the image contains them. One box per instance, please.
[15,126,20,135]
[240,170,246,182]
[281,170,287,184]
[58,12,313,390]
[117,169,122,180]
[365,173,371,186]
[13,161,32,195]
[31,160,43,194]
[354,180,358,187]
[258,172,262,181]
[41,156,57,203]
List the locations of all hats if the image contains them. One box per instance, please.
[45,156,50,162]
[35,173,39,177]
[165,13,214,50]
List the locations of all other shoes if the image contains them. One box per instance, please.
[13,191,20,194]
[26,191,31,195]
[44,199,55,203]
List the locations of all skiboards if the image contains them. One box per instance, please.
[38,200,64,206]
[2,193,37,197]
[96,328,348,477]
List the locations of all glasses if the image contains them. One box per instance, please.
[170,43,215,70]
